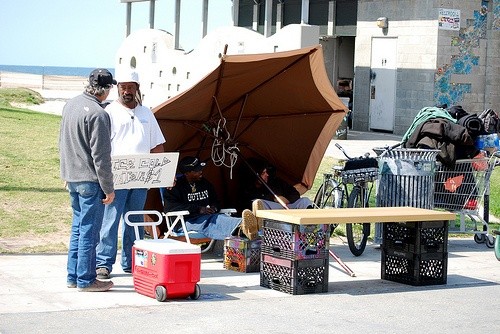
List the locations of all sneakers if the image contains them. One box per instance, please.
[96,268,110,279]
[77,279,113,292]
[67,284,77,288]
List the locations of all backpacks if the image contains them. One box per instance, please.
[400,104,500,210]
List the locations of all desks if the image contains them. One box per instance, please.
[256,206,456,294]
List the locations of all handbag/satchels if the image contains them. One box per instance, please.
[344,158,379,182]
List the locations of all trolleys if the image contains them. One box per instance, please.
[433,149,500,250]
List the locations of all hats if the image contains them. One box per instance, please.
[89,68,117,87]
[117,70,140,85]
[179,156,208,171]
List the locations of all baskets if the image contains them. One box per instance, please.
[342,170,380,184]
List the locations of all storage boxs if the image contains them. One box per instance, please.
[223,237,261,273]
[131,238,200,299]
[260,219,329,294]
[379,221,449,286]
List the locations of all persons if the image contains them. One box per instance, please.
[234,156,313,241]
[58,68,115,292]
[336,81,353,130]
[164,156,265,241]
[96,64,176,280]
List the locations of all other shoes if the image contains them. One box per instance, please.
[252,199,265,231]
[238,209,258,240]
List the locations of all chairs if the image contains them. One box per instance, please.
[158,174,237,253]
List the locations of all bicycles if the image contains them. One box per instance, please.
[312,140,406,257]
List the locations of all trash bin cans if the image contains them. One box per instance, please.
[332,96,350,139]
[373,145,441,248]
